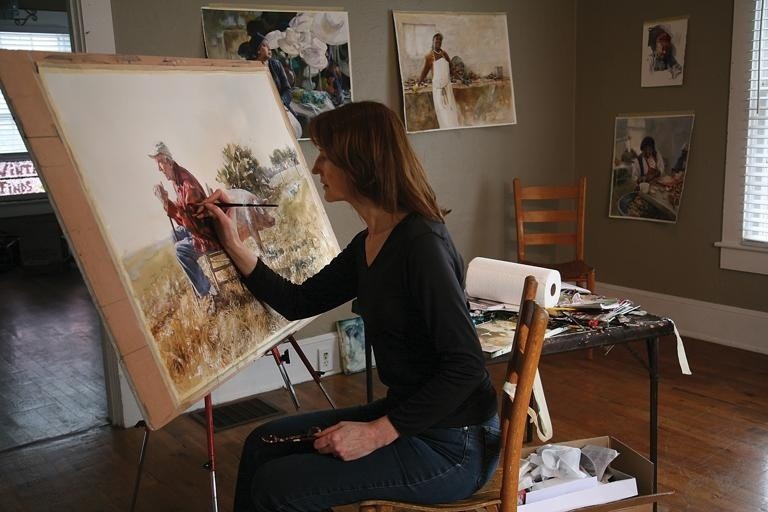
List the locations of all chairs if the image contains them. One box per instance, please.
[359,276,548,512]
[512,177,598,363]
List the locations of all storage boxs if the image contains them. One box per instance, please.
[472,435,675,510]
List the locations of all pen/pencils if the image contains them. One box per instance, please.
[600,299,641,322]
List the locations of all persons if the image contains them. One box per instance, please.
[631,136,665,191]
[146,140,224,316]
[245,30,303,139]
[409,32,461,129]
[647,25,682,78]
[190,101,502,512]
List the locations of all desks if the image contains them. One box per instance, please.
[353,297,674,508]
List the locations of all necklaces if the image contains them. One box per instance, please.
[433,49,442,55]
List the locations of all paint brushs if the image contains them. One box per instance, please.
[186,203,283,207]
[544,307,606,332]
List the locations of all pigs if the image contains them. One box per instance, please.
[225,188,276,255]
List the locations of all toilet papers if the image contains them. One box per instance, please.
[466,257,563,306]
